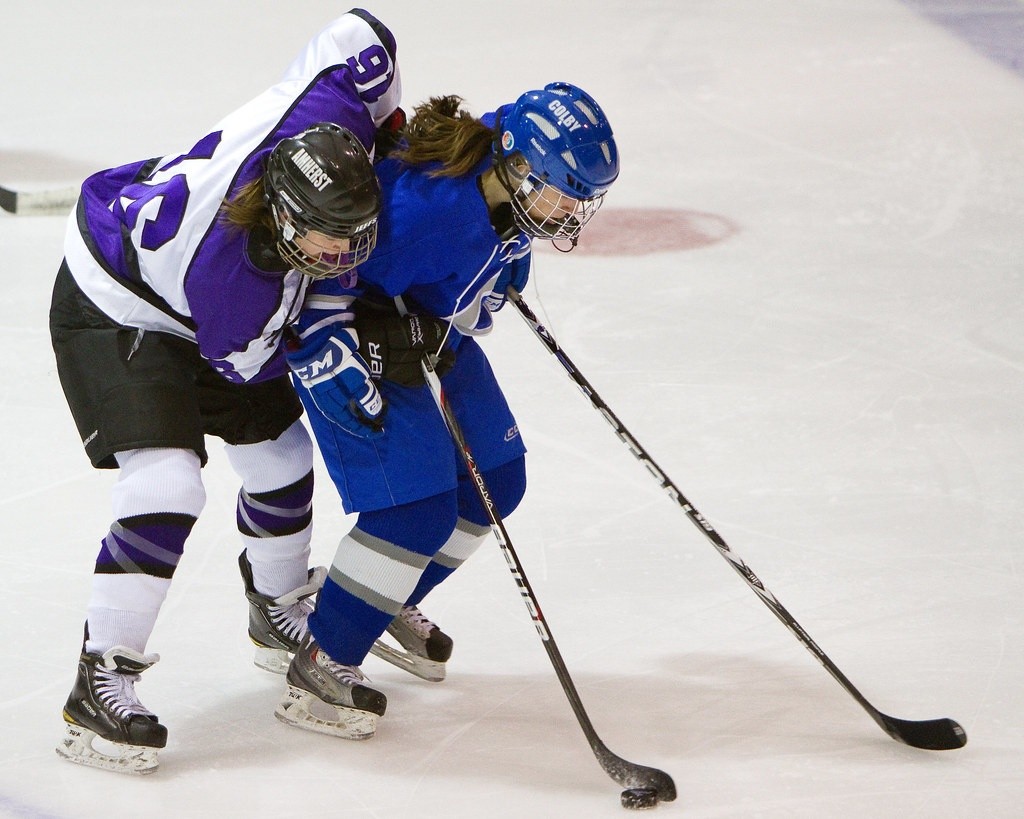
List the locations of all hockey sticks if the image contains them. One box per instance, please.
[390,292,684,813]
[503,285,971,757]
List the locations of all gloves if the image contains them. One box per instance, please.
[346,293,456,388]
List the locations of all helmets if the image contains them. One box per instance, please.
[260,119,384,280]
[480,81,621,253]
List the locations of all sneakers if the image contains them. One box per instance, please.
[238,546,329,675]
[273,626,389,741]
[55,617,168,776]
[368,604,454,683]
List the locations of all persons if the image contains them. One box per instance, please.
[275,82,621,739]
[49,9,406,776]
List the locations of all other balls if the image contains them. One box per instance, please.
[621,786,660,811]
